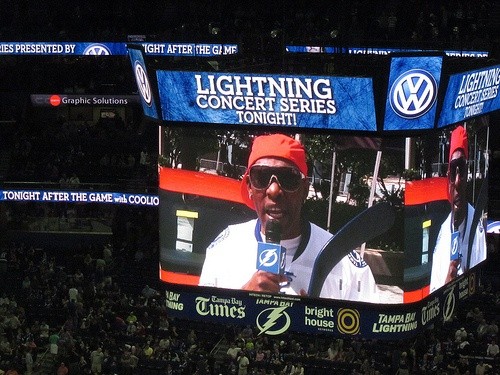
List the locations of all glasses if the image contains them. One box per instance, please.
[449,156,468,179]
[249,164,305,193]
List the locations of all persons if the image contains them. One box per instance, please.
[0,1,500,375]
[198,134,378,303]
[430,126,487,292]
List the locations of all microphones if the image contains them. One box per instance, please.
[256,220,287,275]
[450,210,462,261]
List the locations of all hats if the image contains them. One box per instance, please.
[447,126,468,202]
[239,134,308,211]
